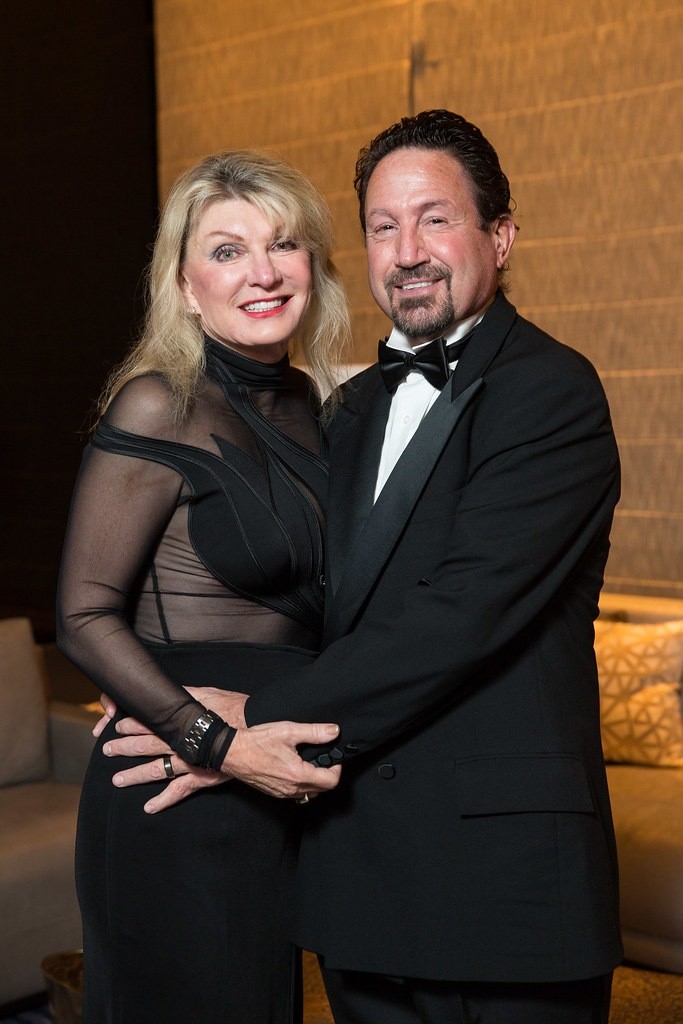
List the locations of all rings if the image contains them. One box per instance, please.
[162,755,176,779]
[295,792,310,805]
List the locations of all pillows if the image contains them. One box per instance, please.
[593,620,683,768]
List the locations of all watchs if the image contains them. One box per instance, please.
[175,708,220,765]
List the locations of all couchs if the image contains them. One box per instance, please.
[0,621,105,1009]
[592,590,683,976]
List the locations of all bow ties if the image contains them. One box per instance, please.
[378,323,480,393]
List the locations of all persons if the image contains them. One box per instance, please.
[60,152,345,1024]
[89,110,621,1023]
